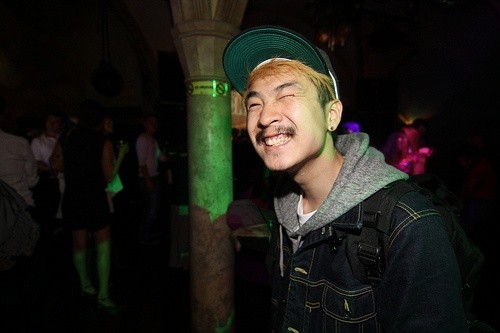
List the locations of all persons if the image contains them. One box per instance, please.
[382,117,428,163]
[29,107,66,209]
[199,25,497,332]
[0,131,39,217]
[47,92,130,314]
[101,110,127,209]
[388,127,430,176]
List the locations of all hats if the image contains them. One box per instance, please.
[224,28,339,99]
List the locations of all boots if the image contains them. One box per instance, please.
[96,242,115,308]
[74,250,95,297]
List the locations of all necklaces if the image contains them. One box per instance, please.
[134,115,164,195]
